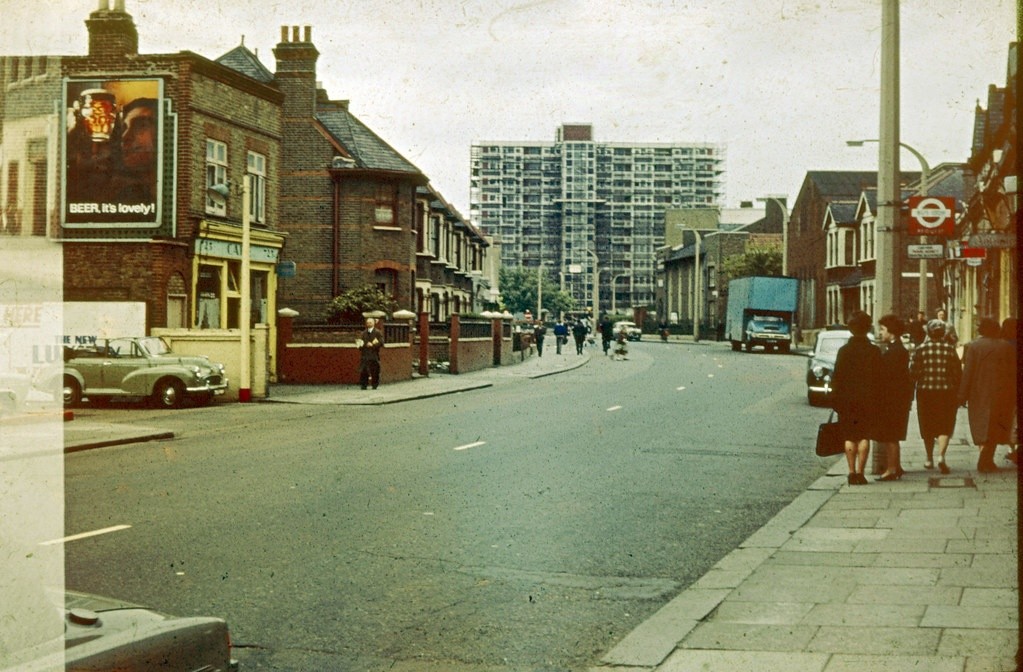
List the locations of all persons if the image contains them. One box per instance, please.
[554,320,586,354]
[68,98,158,206]
[832,311,881,484]
[533,321,546,356]
[717,320,724,341]
[614,335,629,361]
[359,318,384,390]
[910,319,963,469]
[960,318,1018,471]
[873,314,910,480]
[659,319,669,338]
[600,317,614,351]
[794,324,802,348]
[908,309,958,347]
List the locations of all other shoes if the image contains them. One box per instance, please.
[924,460,934,468]
[938,456,950,474]
[874,467,904,481]
[1005,451,1017,460]
[978,452,1001,473]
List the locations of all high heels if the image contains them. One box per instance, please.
[856,473,868,485]
[847,472,855,487]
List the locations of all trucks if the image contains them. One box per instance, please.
[725,277,799,354]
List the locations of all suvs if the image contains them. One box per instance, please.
[613,321,642,341]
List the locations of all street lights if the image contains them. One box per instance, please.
[740,198,789,277]
[572,247,599,335]
[206,175,251,402]
[676,223,702,342]
[596,266,610,338]
[612,274,631,315]
[538,260,554,320]
[847,139,931,320]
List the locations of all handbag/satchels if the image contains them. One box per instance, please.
[816,409,845,457]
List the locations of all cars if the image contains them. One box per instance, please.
[798,328,876,406]
[64,336,230,409]
